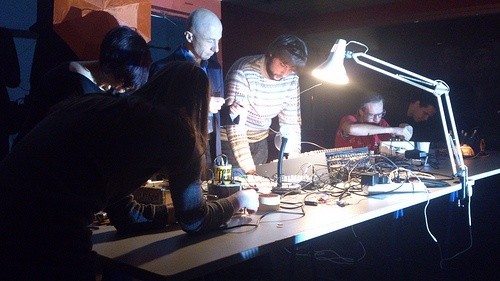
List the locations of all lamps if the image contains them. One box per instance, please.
[308,37,475,200]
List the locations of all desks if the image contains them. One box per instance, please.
[87,144,500,281]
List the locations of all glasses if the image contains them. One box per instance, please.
[362,111,386,119]
[275,44,307,65]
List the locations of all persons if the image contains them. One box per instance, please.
[0,8,260,281]
[220,34,308,175]
[335,92,414,154]
[384,89,439,142]
